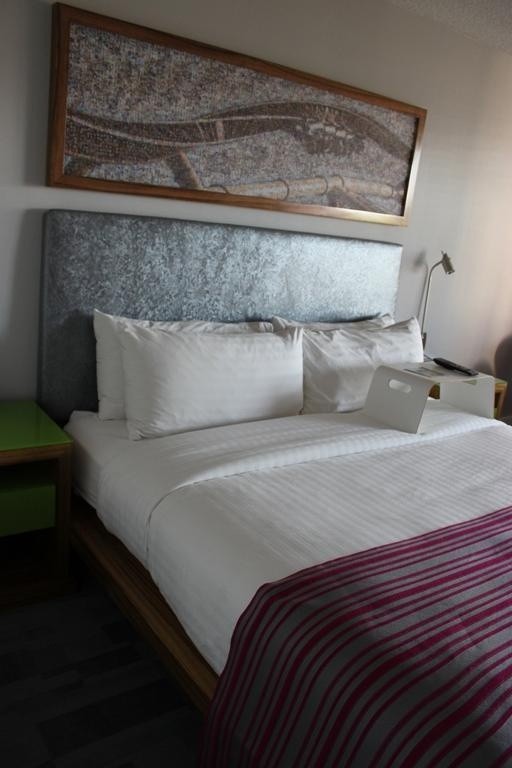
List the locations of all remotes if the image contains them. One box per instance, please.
[432,357,479,376]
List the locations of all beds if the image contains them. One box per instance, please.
[37,209,511,768]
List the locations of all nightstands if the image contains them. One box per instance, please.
[377,360,508,420]
[0,399,75,607]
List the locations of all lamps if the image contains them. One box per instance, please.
[421,250,456,351]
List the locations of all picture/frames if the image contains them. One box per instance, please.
[46,1,431,227]
